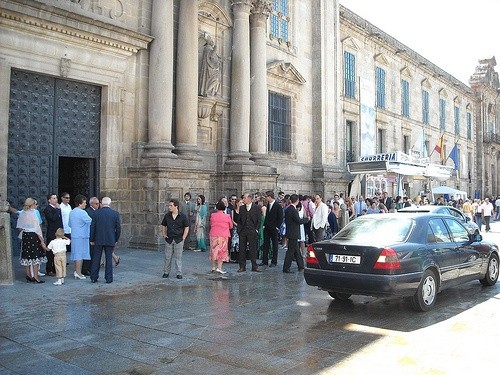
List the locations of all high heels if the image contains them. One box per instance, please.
[74,273,86,279]
[74,272,84,277]
[32,277,45,283]
[26,275,33,283]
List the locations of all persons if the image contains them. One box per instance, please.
[60,192,73,265]
[87,198,120,267]
[90,197,121,283]
[43,228,71,287]
[68,195,92,279]
[9,199,45,276]
[193,195,207,252]
[481,197,494,231]
[44,194,63,277]
[434,195,481,231]
[161,199,190,279]
[179,192,195,251]
[335,192,429,219]
[490,195,500,220]
[209,189,339,274]
[199,35,231,96]
[17,197,45,283]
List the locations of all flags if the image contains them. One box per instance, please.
[450,144,458,170]
[414,131,426,160]
[436,135,444,161]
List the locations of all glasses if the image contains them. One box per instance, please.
[231,198,237,201]
[92,203,99,206]
[265,195,269,198]
[35,203,38,205]
[167,204,174,207]
[63,196,71,200]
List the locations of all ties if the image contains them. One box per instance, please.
[269,201,272,213]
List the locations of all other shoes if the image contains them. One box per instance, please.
[115,256,120,266]
[31,272,34,278]
[163,273,169,278]
[210,239,309,274]
[81,270,91,276]
[47,271,56,276]
[91,279,97,283]
[182,247,185,252]
[38,271,46,277]
[53,281,61,286]
[100,263,104,267]
[177,275,182,279]
[193,248,201,252]
[106,279,113,283]
[61,280,64,284]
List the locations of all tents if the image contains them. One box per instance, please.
[431,186,467,200]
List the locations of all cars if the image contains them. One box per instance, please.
[397,205,480,235]
[303,212,500,313]
